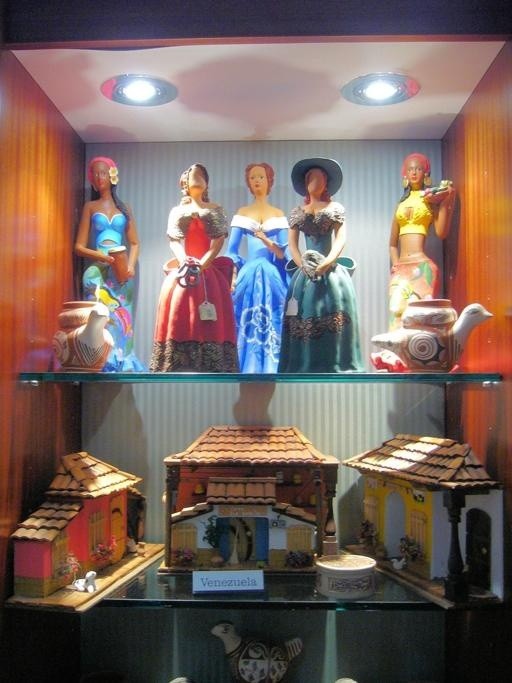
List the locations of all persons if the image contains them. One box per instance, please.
[387,153,456,331]
[74,156,147,372]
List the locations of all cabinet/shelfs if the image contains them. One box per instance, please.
[17,369,505,612]
